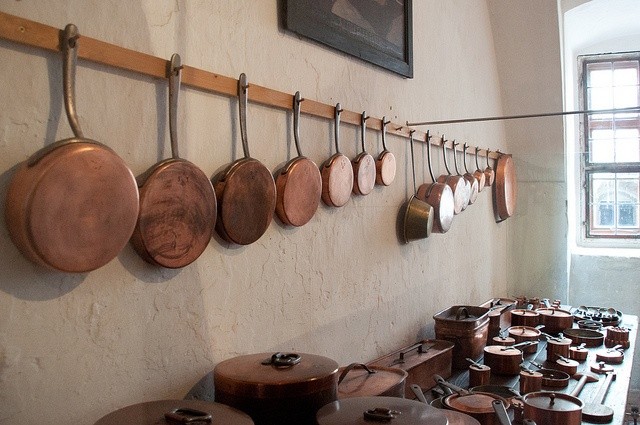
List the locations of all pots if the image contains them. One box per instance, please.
[277,90,322,229]
[462,141,479,206]
[5,21,141,276]
[562,328,605,346]
[465,356,492,388]
[352,109,377,197]
[437,133,466,215]
[518,363,545,395]
[554,351,580,376]
[521,390,586,425]
[482,338,541,376]
[466,383,517,409]
[438,377,511,425]
[541,332,572,361]
[212,72,279,248]
[535,298,573,335]
[485,148,495,187]
[376,115,397,187]
[595,344,625,363]
[494,150,517,223]
[316,395,450,425]
[429,374,453,410]
[492,328,516,347]
[509,303,540,328]
[416,129,455,236]
[451,138,471,211]
[129,52,220,272]
[92,397,258,425]
[529,359,571,388]
[321,102,355,209]
[507,324,546,354]
[212,350,340,423]
[473,145,486,194]
[569,342,590,360]
[402,130,435,246]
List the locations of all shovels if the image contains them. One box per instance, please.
[569,370,599,397]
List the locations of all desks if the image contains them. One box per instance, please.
[415,303,640,424]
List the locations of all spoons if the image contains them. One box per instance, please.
[588,307,599,318]
[608,306,616,321]
[599,307,608,320]
[577,304,587,318]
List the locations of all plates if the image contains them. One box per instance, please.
[573,305,624,325]
